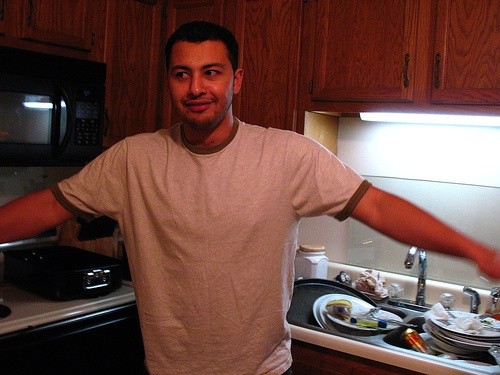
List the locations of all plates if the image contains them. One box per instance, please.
[312,293,403,336]
[420,311,500,358]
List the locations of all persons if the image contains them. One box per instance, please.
[0,20,500,375]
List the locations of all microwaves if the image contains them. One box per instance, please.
[0,45,107,168]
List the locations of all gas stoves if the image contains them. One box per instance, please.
[0,194,135,332]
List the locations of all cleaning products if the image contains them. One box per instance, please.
[484,287,500,321]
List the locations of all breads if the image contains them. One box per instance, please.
[326,300,352,321]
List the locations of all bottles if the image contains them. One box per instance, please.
[294,245,329,280]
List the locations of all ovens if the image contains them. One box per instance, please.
[0,303,148,375]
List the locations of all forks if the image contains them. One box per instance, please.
[370,311,418,329]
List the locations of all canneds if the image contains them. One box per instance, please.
[400,327,434,355]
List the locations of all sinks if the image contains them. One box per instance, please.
[404,245,427,306]
[378,307,500,375]
[290,294,412,342]
